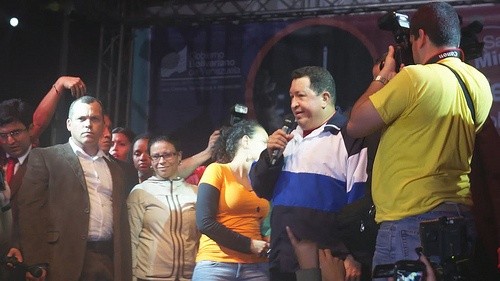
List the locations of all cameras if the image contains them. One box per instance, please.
[378,10,416,73]
[220,105,249,136]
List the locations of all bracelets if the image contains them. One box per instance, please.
[373,74,389,85]
[52,84,61,96]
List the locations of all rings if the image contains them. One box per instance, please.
[267,249,272,254]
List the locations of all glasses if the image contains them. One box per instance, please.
[0,127,27,139]
[148,153,175,161]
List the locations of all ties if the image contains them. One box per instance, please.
[5,158,15,185]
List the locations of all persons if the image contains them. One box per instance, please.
[0,67,436,281]
[346,1,493,281]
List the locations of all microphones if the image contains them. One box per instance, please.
[269,114,296,165]
[4,256,43,277]
